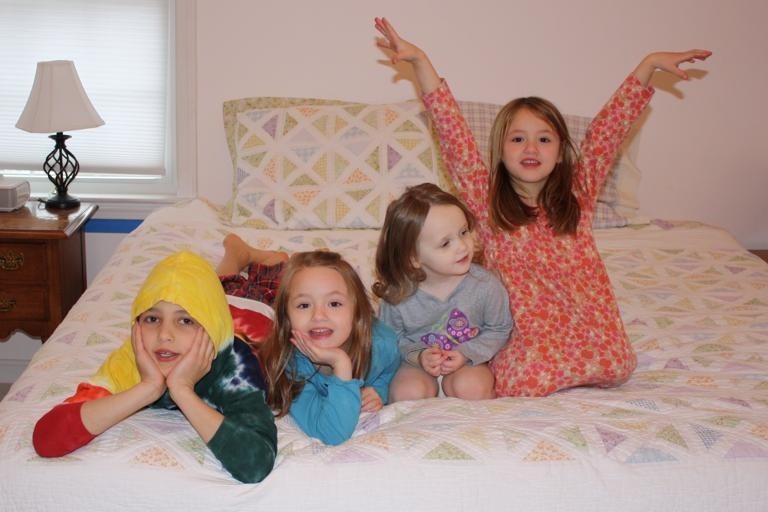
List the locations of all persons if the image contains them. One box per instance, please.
[374,15,712,397]
[261,250,403,444]
[33,233,289,484]
[370,182,514,403]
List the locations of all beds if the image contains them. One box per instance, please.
[0,200,768,512]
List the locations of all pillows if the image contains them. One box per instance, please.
[223,96,456,229]
[458,102,651,228]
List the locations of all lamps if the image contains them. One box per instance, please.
[15,61,106,209]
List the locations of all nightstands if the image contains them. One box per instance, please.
[0,199,99,344]
